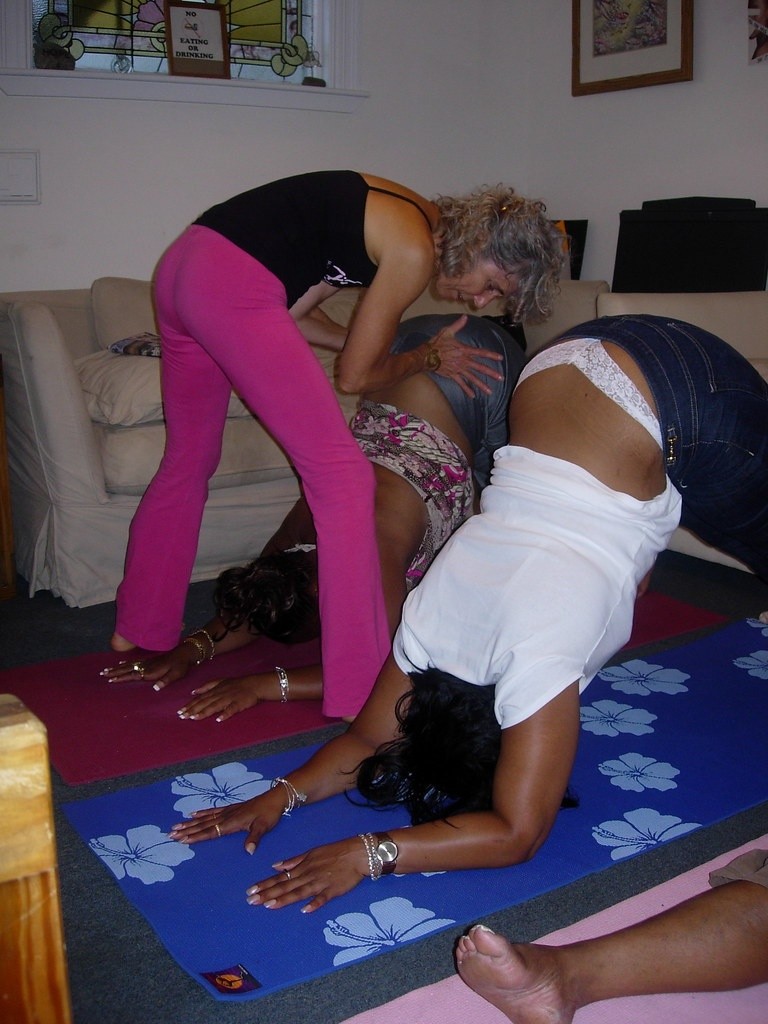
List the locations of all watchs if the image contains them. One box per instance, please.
[414,342,441,372]
[371,831,398,877]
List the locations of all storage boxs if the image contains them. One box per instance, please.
[0,693,74,1024]
[610,197,768,293]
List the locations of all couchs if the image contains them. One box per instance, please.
[0,276,768,606]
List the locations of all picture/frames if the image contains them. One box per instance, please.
[570,0,694,96]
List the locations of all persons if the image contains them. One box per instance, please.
[171,314,768,916]
[455,849,768,1024]
[101,314,517,721]
[105,170,571,726]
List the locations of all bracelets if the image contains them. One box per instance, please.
[190,629,216,660]
[184,638,206,666]
[359,832,381,880]
[270,777,306,817]
[274,668,289,701]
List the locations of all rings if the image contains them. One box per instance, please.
[215,824,222,836]
[212,811,215,818]
[133,663,146,678]
[283,868,293,879]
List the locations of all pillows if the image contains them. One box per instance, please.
[73,346,364,427]
